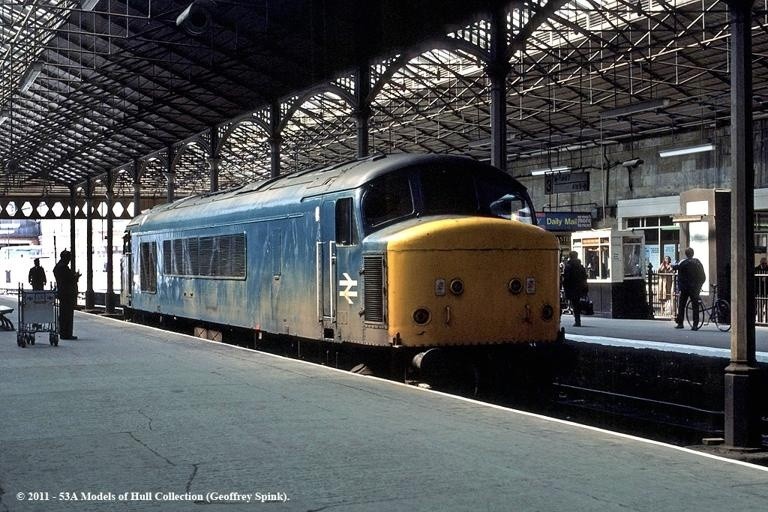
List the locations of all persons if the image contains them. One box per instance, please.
[564,250,587,327]
[657,256,674,315]
[28,258,47,290]
[755,258,768,315]
[669,247,706,331]
[52,250,81,339]
[559,252,610,280]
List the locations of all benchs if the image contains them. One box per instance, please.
[0,304,14,329]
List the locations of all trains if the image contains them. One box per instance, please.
[118,148,564,394]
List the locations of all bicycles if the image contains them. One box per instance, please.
[685,283,731,331]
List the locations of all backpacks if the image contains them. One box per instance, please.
[570,262,590,296]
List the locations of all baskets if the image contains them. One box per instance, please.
[580,299,594,316]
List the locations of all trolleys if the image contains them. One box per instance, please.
[15,281,61,347]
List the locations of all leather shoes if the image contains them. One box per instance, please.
[572,322,582,327]
[60,335,78,340]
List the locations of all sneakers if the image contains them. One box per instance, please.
[675,324,684,328]
[691,326,698,331]
